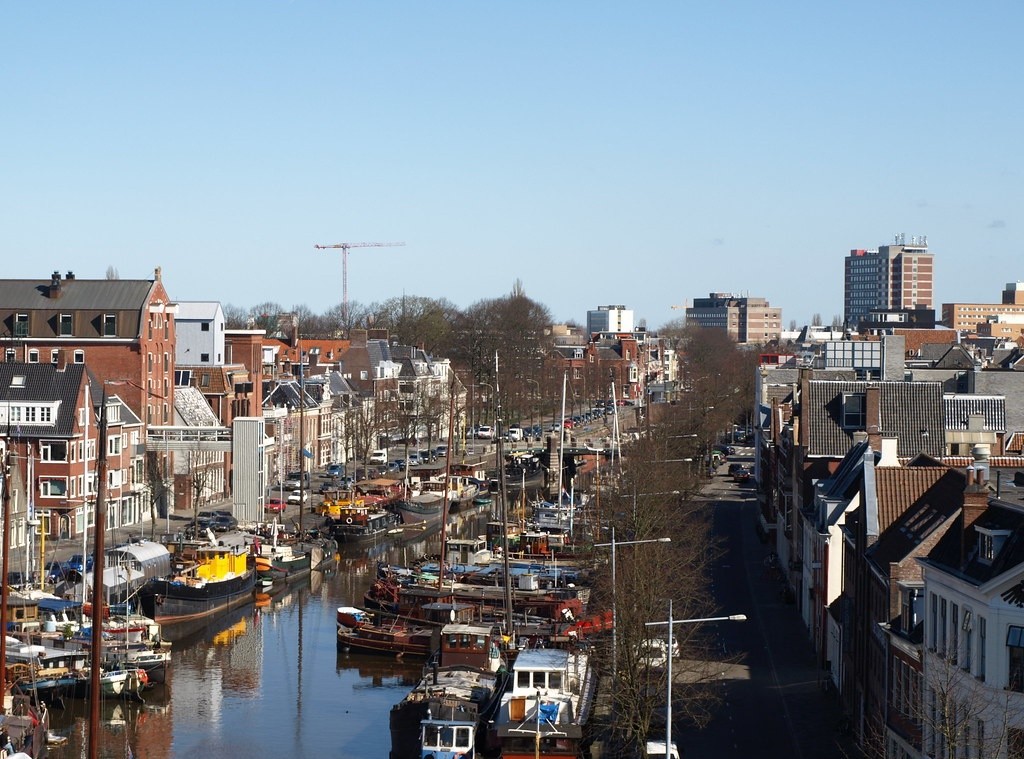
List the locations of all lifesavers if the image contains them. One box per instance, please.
[346,517,352,523]
[138,670,148,682]
[294,522,300,533]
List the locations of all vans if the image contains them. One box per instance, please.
[477,425,494,440]
[370,450,388,465]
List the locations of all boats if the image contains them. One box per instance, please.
[1,345,624,757]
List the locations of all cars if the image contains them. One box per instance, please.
[195,510,219,527]
[287,490,307,505]
[700,429,750,482]
[376,465,388,477]
[187,520,211,537]
[68,553,93,572]
[319,480,338,495]
[436,446,449,457]
[393,459,405,471]
[264,498,286,514]
[409,454,423,466]
[339,474,355,488]
[7,571,31,590]
[45,560,70,583]
[387,462,400,474]
[210,514,239,533]
[326,463,344,478]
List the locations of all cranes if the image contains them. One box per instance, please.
[314,240,407,327]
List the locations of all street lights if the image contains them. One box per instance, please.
[662,432,697,473]
[621,482,680,611]
[643,598,747,759]
[527,377,540,396]
[479,382,495,420]
[593,528,673,680]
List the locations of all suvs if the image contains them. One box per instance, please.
[638,634,680,672]
[285,472,309,492]
